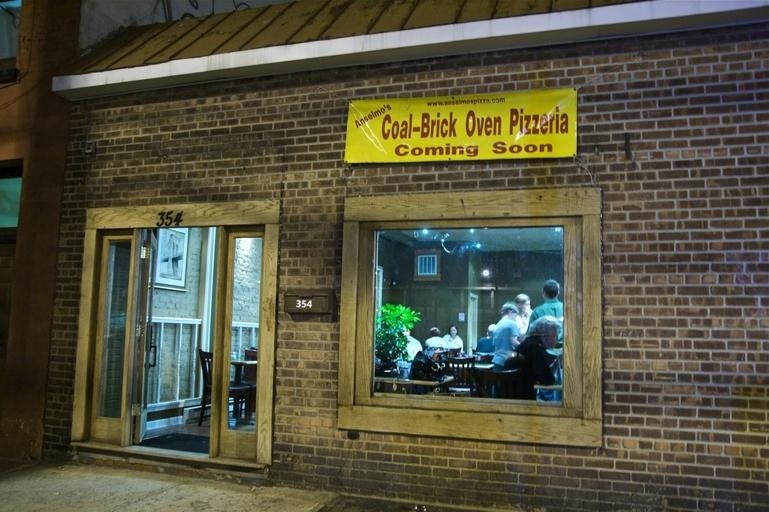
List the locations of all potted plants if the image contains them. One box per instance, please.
[374,304,421,377]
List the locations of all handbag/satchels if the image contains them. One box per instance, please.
[408,351,449,383]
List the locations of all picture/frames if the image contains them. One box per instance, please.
[148,228,191,291]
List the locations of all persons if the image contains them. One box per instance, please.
[399,328,424,361]
[502,313,562,400]
[424,326,449,349]
[511,292,535,334]
[440,324,465,354]
[524,278,564,344]
[474,323,497,352]
[489,303,521,371]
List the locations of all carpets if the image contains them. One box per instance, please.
[139,433,209,453]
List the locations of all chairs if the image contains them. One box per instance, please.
[199,349,251,426]
[443,348,523,399]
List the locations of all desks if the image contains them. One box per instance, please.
[230,360,257,418]
[375,376,454,394]
